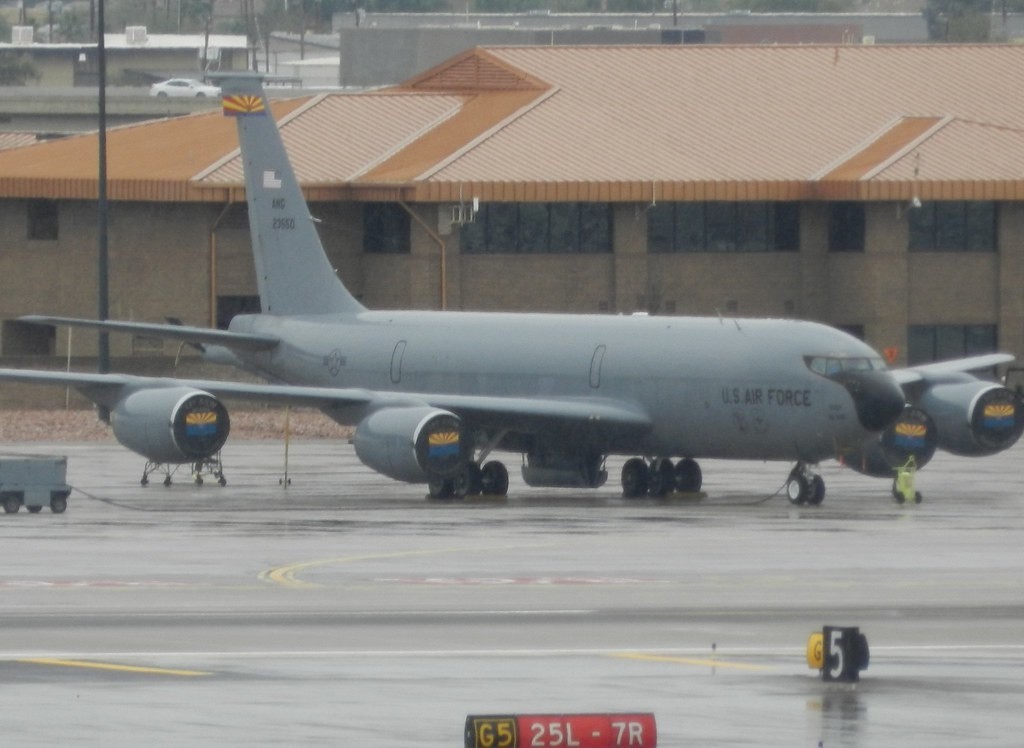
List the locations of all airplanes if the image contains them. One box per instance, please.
[0,77,1023,505]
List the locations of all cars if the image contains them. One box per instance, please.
[151,79,222,99]
